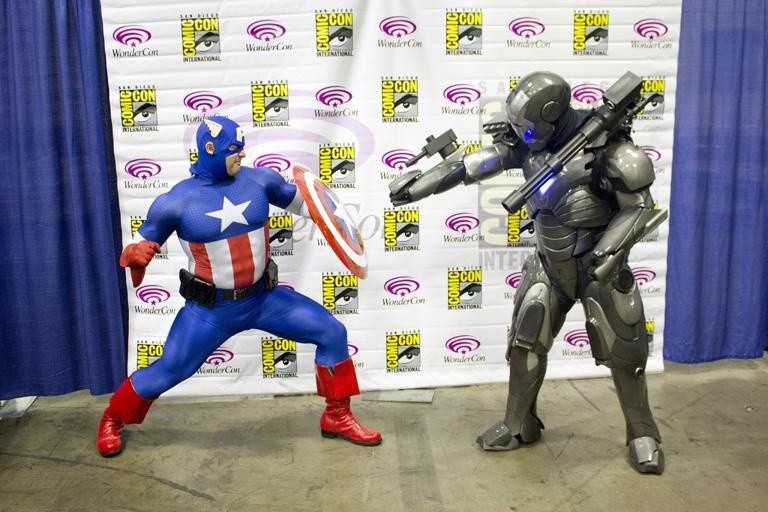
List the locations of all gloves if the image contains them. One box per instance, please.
[119,240,162,270]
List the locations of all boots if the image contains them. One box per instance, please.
[320,398,383,447]
[97,406,125,457]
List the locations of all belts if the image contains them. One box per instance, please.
[223,281,258,301]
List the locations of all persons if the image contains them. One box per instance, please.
[95,114,382,457]
[388,68,665,479]
